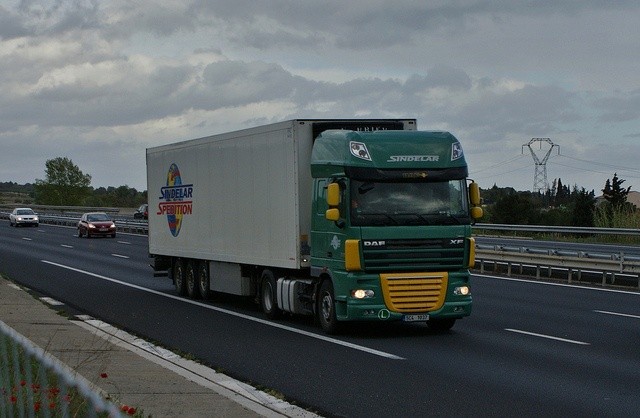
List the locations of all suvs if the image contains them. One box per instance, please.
[133,203,148,219]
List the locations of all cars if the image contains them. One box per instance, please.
[77,212,116,238]
[9,208,39,227]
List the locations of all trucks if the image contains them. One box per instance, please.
[146,118,484,332]
